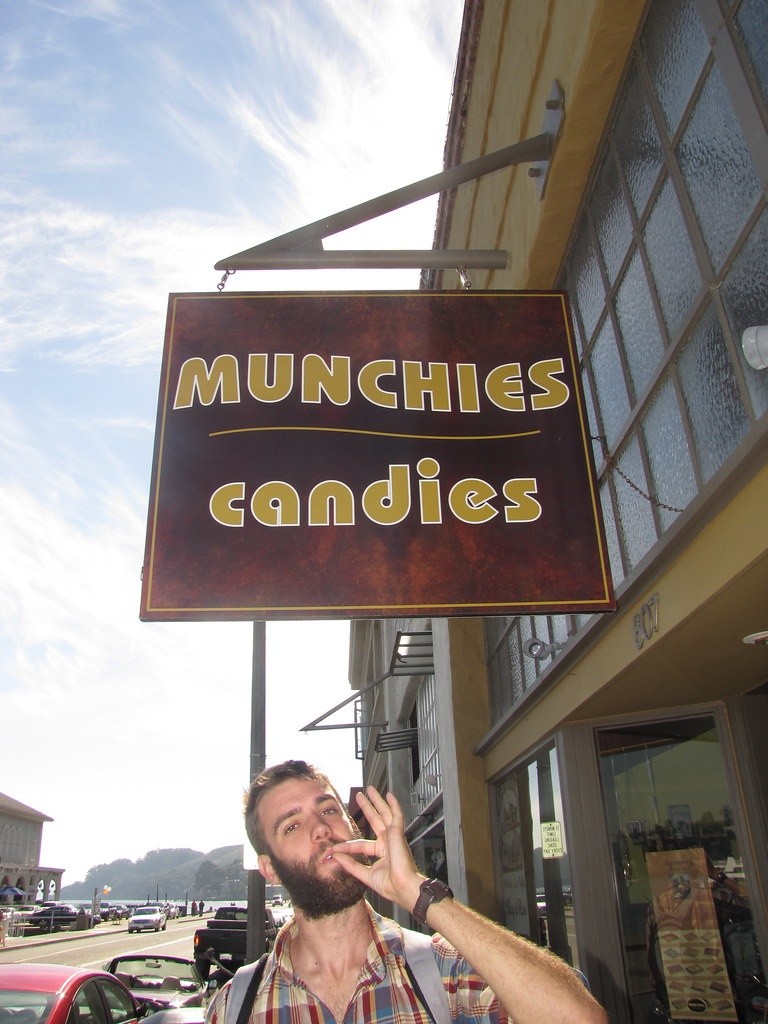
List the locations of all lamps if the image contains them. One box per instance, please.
[424,772,442,787]
[411,792,425,805]
[741,325,768,370]
[522,637,568,661]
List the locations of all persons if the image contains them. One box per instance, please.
[165,903,169,919]
[646,842,752,1024]
[192,900,196,917]
[79,907,86,915]
[205,759,608,1024]
[0,910,7,932]
[199,900,204,916]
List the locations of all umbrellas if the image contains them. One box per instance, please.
[0,885,28,904]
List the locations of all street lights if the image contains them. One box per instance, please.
[90,885,111,928]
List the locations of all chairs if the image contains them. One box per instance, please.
[159,976,183,992]
[115,972,144,988]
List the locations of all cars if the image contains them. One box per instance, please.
[150,902,179,920]
[17,905,43,920]
[29,905,101,928]
[271,895,283,907]
[562,891,572,906]
[76,902,113,922]
[536,895,547,916]
[107,954,217,1017]
[109,905,131,920]
[0,963,207,1024]
[0,908,21,926]
[128,907,167,933]
[39,901,66,910]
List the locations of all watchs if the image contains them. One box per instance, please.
[412,877,454,928]
[717,872,727,883]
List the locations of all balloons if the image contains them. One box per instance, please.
[104,885,111,894]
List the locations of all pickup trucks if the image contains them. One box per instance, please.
[193,906,278,977]
